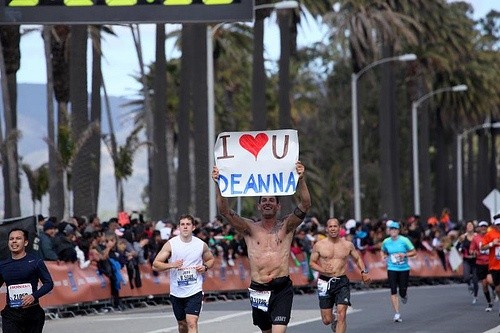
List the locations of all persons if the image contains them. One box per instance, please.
[0,228,54,333]
[480,212,500,317]
[468,221,499,312]
[152,214,215,333]
[211,161,312,332]
[36,208,466,287]
[380,222,416,323]
[310,215,370,333]
[454,221,480,305]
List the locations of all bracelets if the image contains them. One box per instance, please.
[360,270,368,274]
[203,264,208,271]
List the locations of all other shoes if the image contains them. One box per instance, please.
[493,290,499,302]
[330,311,337,332]
[472,296,478,305]
[401,291,408,304]
[393,313,402,323]
[485,303,493,312]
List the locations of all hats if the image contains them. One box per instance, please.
[390,222,399,228]
[479,221,489,226]
[44,221,56,231]
[494,218,500,226]
[38,214,48,221]
